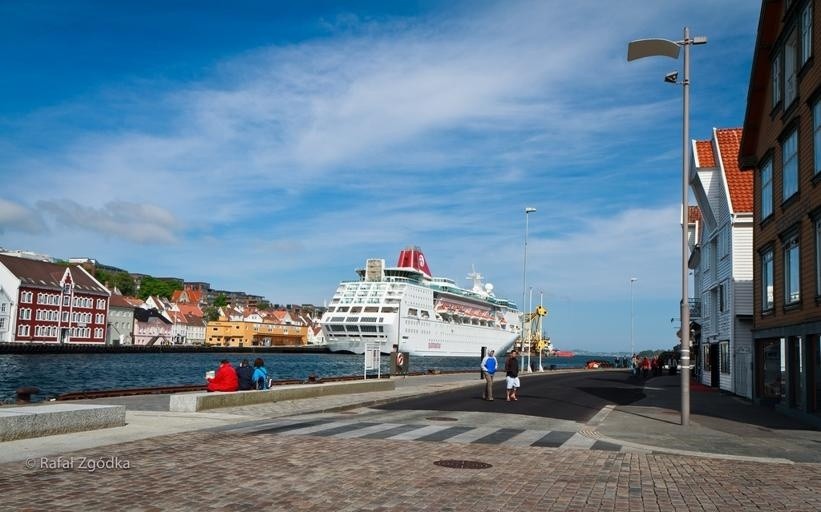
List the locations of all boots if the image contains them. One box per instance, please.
[482,383,494,401]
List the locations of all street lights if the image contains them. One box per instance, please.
[540,287,546,373]
[626,25,710,423]
[629,277,639,356]
[527,285,534,373]
[518,208,536,373]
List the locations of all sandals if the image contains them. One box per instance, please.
[506,395,518,401]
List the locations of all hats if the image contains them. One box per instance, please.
[488,349,494,354]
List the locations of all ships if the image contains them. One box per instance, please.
[317,247,524,359]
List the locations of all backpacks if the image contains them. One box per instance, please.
[257,368,273,391]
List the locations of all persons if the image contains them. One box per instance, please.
[505,350,520,402]
[480,348,498,401]
[236,360,254,389]
[251,357,270,390]
[207,359,239,393]
[587,353,678,378]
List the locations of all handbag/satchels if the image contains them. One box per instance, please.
[633,363,636,368]
